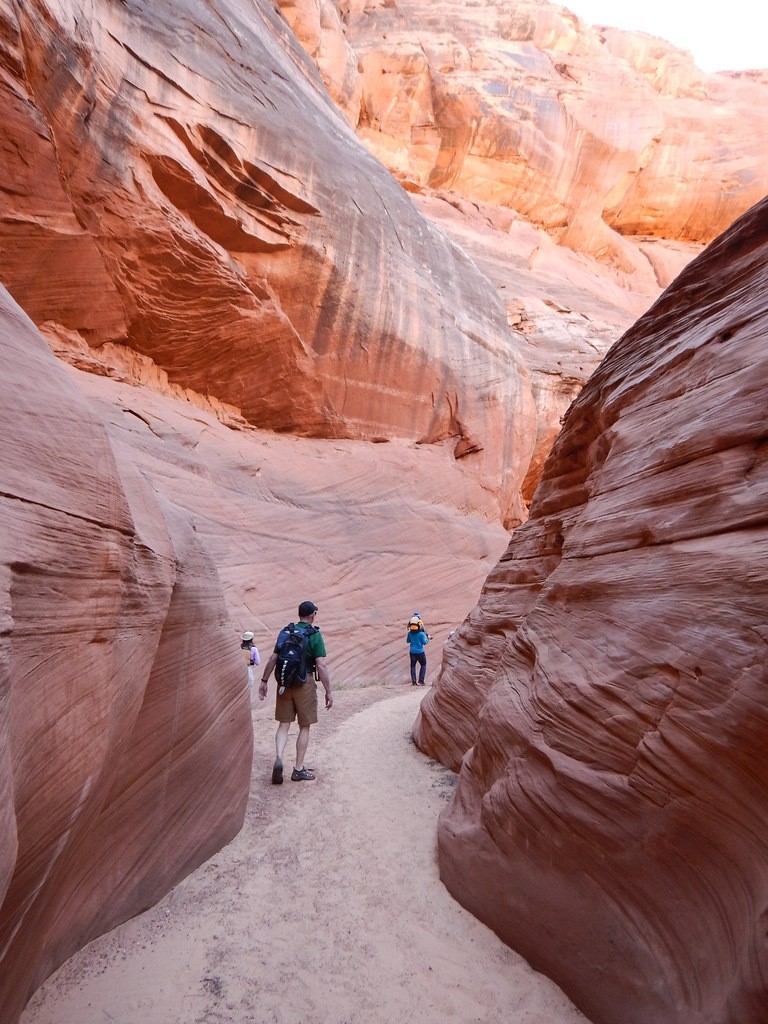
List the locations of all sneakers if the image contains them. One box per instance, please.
[272,755,283,784]
[291,765,315,781]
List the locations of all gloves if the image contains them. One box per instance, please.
[428,635,430,640]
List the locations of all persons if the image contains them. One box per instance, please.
[407,611,433,640]
[238,632,260,687]
[258,601,334,784]
[406,629,430,685]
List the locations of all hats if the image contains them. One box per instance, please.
[299,601,318,616]
[410,616,419,624]
[450,631,455,636]
[241,632,254,641]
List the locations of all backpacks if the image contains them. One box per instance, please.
[241,644,254,666]
[275,623,319,686]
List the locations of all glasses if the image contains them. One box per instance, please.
[315,612,316,615]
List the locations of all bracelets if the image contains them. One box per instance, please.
[261,679,267,683]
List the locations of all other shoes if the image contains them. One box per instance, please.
[418,681,425,684]
[413,683,417,685]
